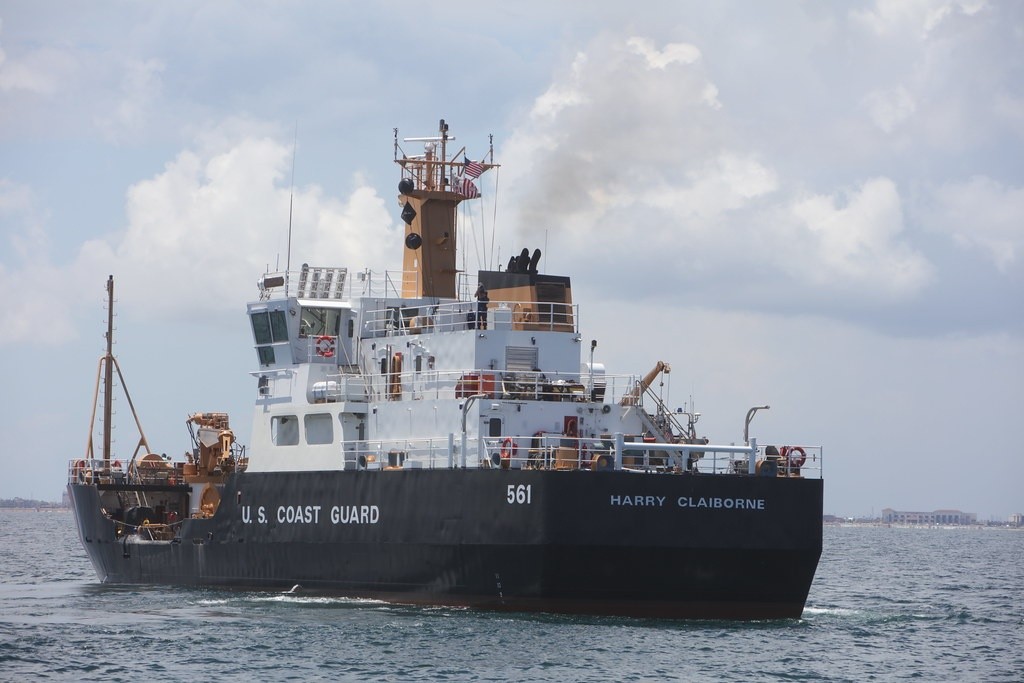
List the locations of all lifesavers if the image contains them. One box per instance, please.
[316,336,335,357]
[785,447,806,467]
[501,438,513,458]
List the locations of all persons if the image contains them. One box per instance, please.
[474,281,490,329]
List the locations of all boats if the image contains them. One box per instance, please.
[67,120,823,620]
